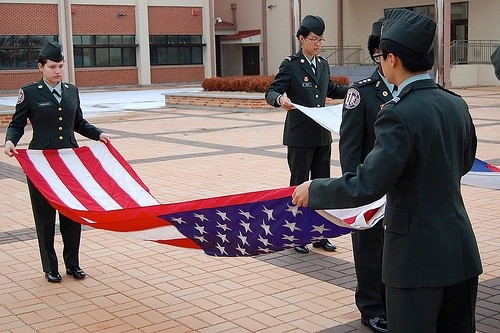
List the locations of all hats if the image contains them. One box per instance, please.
[39,39,64,63]
[300,15,325,36]
[371,16,385,37]
[380,9,437,55]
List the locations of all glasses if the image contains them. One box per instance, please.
[373,52,400,62]
[302,35,325,43]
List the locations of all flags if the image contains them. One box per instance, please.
[290,100,500,189]
[10,139,386,257]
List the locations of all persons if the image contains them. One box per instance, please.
[291,9,483,333]
[265,15,352,254]
[5,39,111,283]
[339,18,399,333]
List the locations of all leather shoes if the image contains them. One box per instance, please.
[294,245,309,254]
[361,314,387,333]
[66,266,86,279]
[45,270,62,283]
[313,239,337,251]
